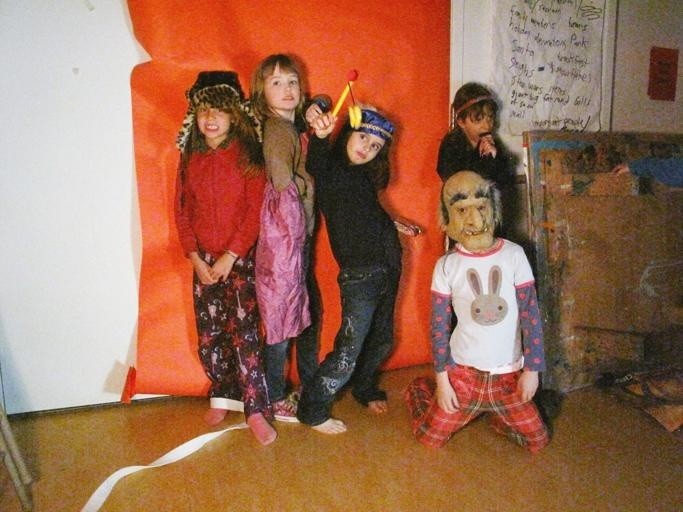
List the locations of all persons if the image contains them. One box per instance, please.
[174,66,278,443]
[292,104,422,434]
[434,80,524,335]
[249,53,323,425]
[399,168,550,454]
[611,158,683,188]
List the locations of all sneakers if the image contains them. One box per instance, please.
[270,398,300,422]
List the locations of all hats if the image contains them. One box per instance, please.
[190,71,244,112]
[353,109,393,140]
